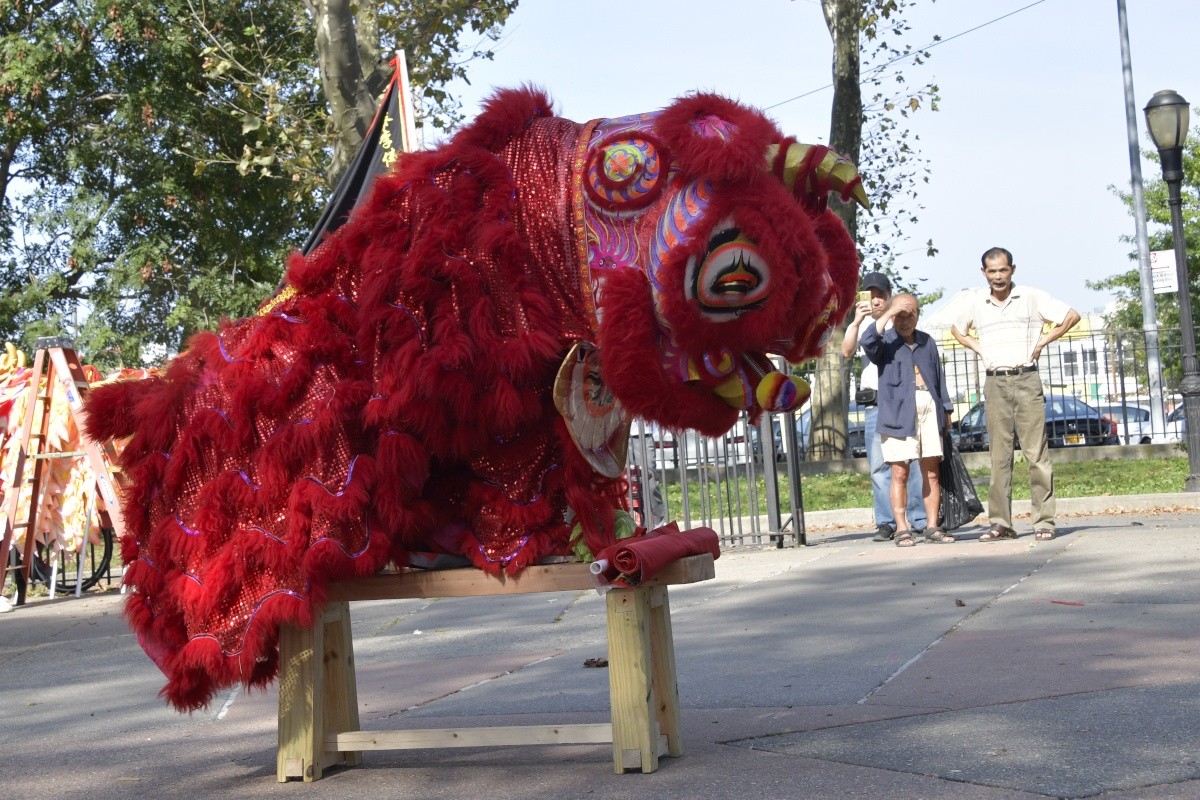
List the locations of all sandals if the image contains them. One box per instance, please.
[1034,528,1055,540]
[979,523,1016,541]
[893,531,915,546]
[922,527,954,544]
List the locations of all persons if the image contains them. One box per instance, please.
[841,273,954,547]
[950,246,1081,541]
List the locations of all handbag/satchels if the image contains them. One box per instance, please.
[938,427,985,531]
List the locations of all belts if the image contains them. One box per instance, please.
[986,366,1037,376]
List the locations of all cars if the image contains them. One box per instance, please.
[778,398,867,465]
[1166,401,1189,443]
[950,395,1121,453]
[1088,402,1152,444]
[746,420,785,462]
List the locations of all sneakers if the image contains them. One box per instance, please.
[872,524,894,541]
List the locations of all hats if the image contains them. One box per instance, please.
[864,272,891,291]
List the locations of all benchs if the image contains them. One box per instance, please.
[276,551,715,784]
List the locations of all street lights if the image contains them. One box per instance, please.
[1142,85,1200,492]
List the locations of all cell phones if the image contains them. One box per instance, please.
[860,291,872,310]
